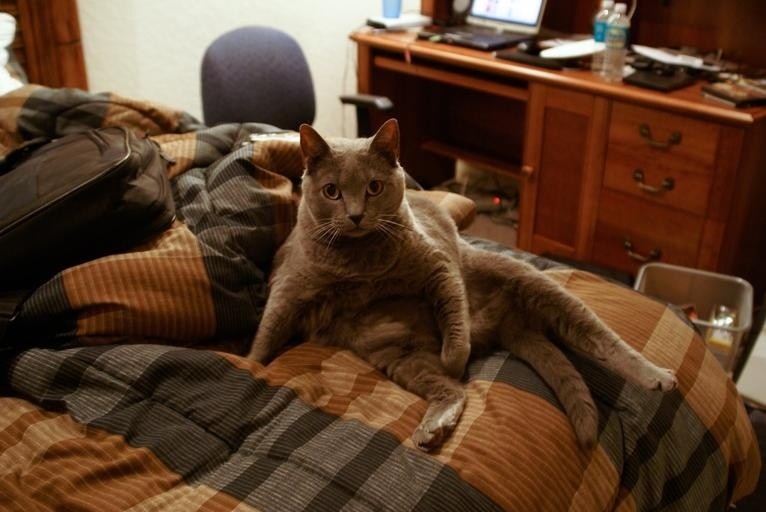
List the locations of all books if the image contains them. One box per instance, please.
[701,76,766,109]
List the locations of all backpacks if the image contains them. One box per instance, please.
[0,125,175,291]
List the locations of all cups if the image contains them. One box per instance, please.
[382,0,402,17]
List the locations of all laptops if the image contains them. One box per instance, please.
[418,0,547,51]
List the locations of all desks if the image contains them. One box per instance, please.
[348,14,766,280]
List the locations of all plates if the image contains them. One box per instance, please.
[539,38,593,60]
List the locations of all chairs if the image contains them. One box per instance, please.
[200,26,394,130]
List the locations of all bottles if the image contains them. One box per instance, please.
[591,0,630,83]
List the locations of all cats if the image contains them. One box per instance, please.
[244,117,681,454]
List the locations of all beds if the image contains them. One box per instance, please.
[1,87,762,510]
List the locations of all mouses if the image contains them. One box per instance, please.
[517,40,541,55]
